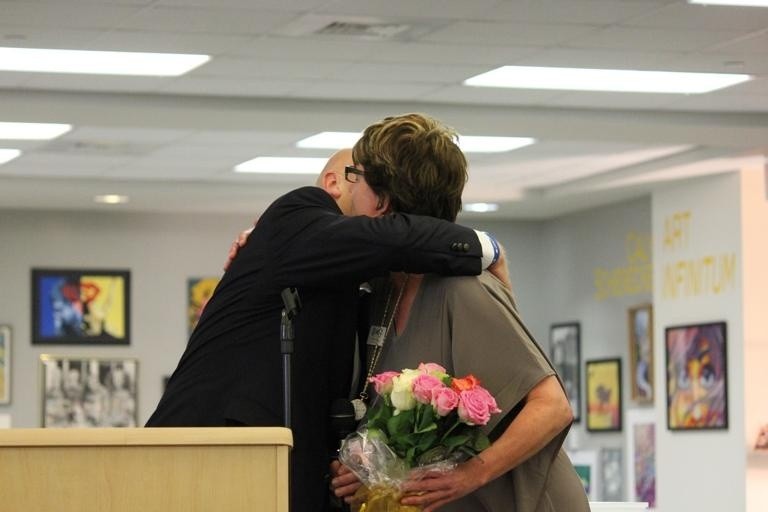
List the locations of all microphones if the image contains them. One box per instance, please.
[331,397,354,456]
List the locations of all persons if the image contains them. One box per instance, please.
[223,114,590,512]
[144,148,512,512]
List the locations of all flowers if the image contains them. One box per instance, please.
[355,360,503,470]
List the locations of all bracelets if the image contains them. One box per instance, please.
[482,230,501,268]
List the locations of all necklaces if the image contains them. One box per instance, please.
[350,272,410,422]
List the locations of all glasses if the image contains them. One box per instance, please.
[343,164,366,184]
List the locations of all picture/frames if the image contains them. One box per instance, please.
[38,353,141,429]
[29,267,130,347]
[549,321,582,423]
[0,324,11,405]
[584,357,622,433]
[626,304,654,402]
